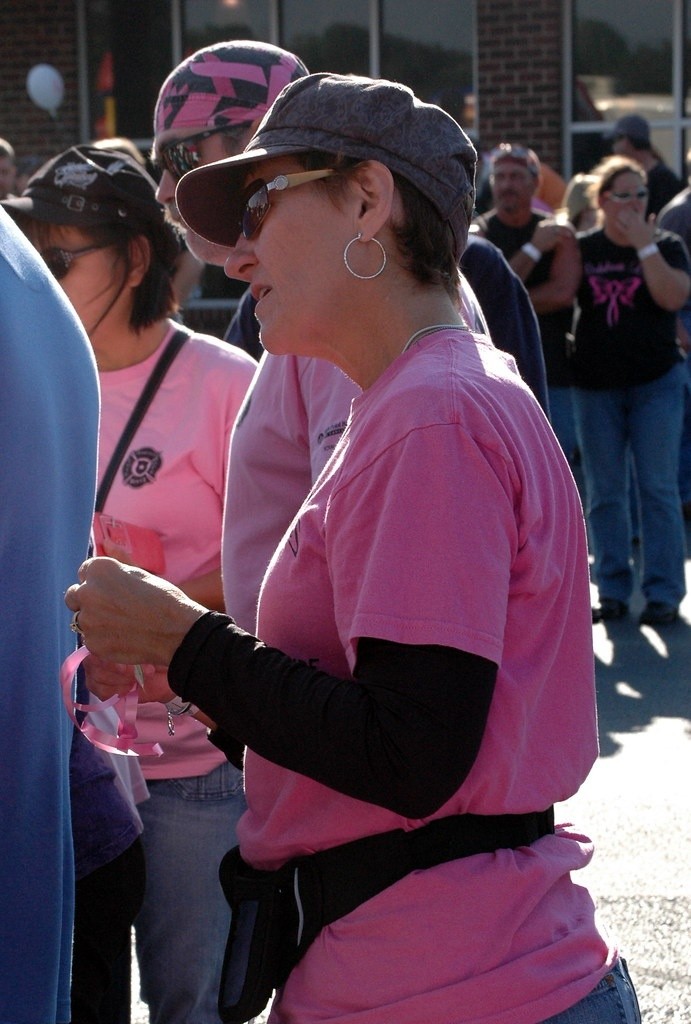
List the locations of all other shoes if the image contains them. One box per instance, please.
[638,601,677,626]
[600,597,627,621]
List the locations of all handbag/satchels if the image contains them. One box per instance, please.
[217,805,555,1024]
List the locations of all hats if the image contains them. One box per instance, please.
[175,72,478,267]
[602,115,648,143]
[0,145,166,234]
[490,144,540,177]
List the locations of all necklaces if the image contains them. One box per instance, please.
[400,323,469,353]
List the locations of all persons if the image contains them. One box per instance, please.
[0,41,691,1024]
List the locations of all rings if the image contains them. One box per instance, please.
[70,611,85,637]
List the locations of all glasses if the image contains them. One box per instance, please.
[236,168,338,240]
[153,124,251,181]
[38,244,101,286]
[606,191,647,202]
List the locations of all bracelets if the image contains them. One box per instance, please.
[165,692,200,735]
[521,243,541,261]
[636,243,658,261]
